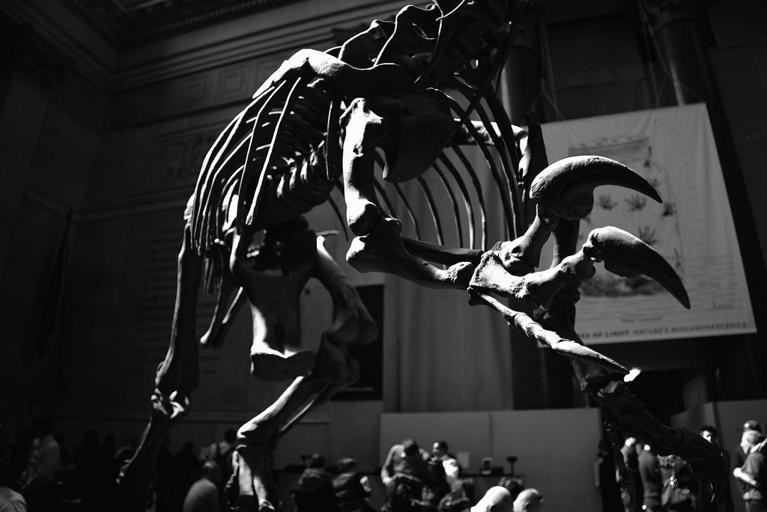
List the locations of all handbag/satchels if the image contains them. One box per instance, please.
[661,482,691,510]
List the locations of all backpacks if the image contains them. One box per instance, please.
[213,443,236,470]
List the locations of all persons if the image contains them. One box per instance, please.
[9,421,239,512]
[293,437,541,512]
[592,418,767,512]
